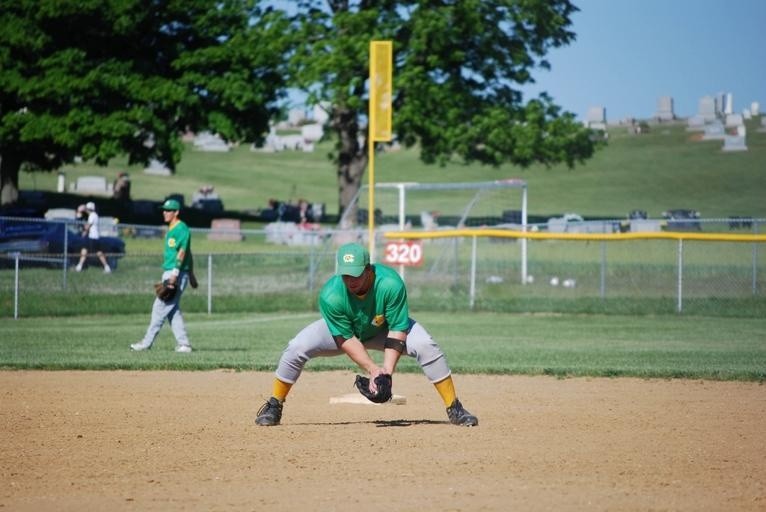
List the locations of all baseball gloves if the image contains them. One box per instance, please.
[154,279,179,305]
[356,372,392,403]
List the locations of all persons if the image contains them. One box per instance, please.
[253,240,482,428]
[114,171,131,201]
[256,197,281,221]
[70,201,111,275]
[205,184,221,201]
[190,186,207,210]
[128,198,198,353]
[109,173,124,200]
[286,198,314,225]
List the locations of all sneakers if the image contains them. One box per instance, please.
[447,398,478,427]
[70,266,79,271]
[175,345,191,354]
[255,397,282,426]
[130,341,150,351]
[102,270,111,273]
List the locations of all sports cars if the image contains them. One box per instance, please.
[0,207,126,269]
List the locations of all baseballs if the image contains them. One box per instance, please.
[375,390,379,393]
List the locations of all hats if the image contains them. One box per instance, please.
[337,243,370,278]
[157,199,179,210]
[86,202,94,210]
